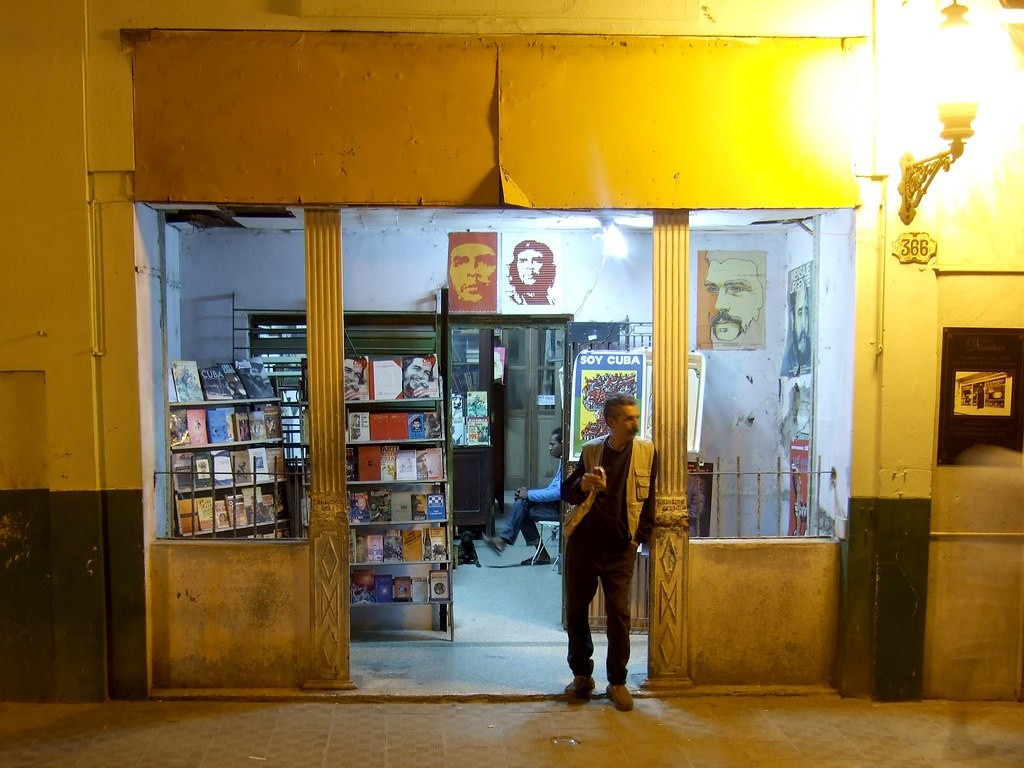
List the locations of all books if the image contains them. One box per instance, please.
[451,341,492,447]
[169,353,449,604]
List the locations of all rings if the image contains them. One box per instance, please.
[591,486,594,490]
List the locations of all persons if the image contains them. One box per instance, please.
[412,418,421,431]
[239,419,249,441]
[250,361,273,399]
[218,513,229,526]
[351,498,370,522]
[266,418,275,437]
[469,397,485,417]
[792,463,807,536]
[425,414,441,438]
[403,355,439,399]
[344,354,368,402]
[237,461,246,479]
[686,475,706,537]
[246,495,265,524]
[560,394,657,711]
[351,414,360,439]
[781,276,811,377]
[482,427,564,566]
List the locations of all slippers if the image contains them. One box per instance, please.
[481,532,501,557]
[521,556,551,565]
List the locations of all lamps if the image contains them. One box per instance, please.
[899,1,982,225]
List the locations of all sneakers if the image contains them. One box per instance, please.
[563,674,596,697]
[606,681,634,711]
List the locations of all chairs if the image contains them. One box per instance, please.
[532,521,560,572]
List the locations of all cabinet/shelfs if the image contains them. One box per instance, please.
[452,329,510,538]
[170,373,456,643]
